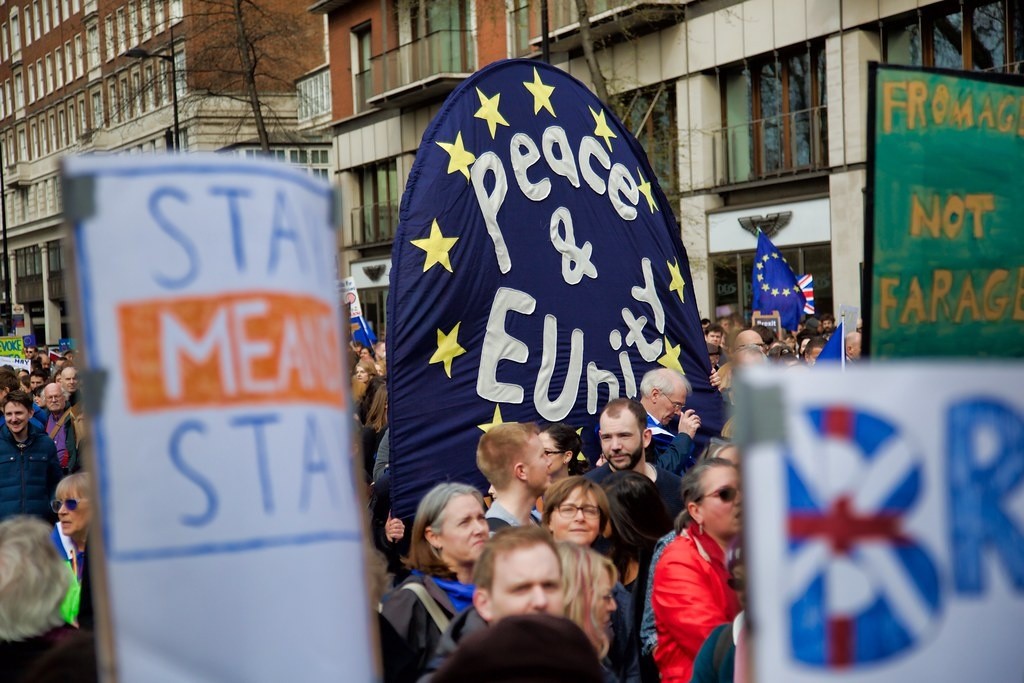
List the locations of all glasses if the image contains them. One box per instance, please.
[544,451,565,456]
[660,389,686,408]
[694,487,743,504]
[553,504,601,518]
[50,499,90,513]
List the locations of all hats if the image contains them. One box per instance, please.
[706,343,718,355]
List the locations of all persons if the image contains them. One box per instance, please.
[0,316,864,683]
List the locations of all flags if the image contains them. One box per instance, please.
[351,317,378,349]
[753,232,847,366]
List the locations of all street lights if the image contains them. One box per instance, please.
[123,25,180,153]
